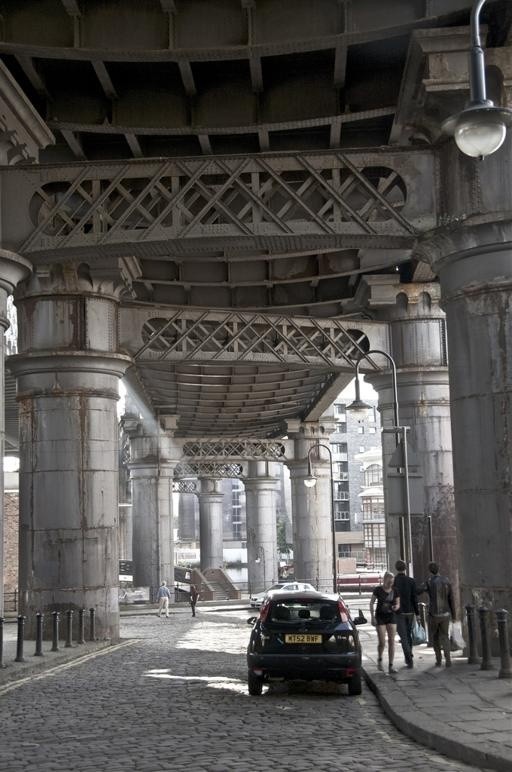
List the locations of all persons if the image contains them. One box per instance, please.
[188,584,200,617]
[391,558,418,671]
[368,571,401,671]
[416,561,455,668]
[156,580,171,618]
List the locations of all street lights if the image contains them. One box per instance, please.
[302,444,337,593]
[344,349,406,577]
[441,0,512,162]
[254,546,266,592]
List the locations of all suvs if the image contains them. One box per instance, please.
[247,593,367,696]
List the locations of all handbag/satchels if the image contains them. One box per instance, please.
[450,627,466,651]
[411,623,426,645]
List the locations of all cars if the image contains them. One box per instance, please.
[249,582,316,612]
[279,565,295,580]
